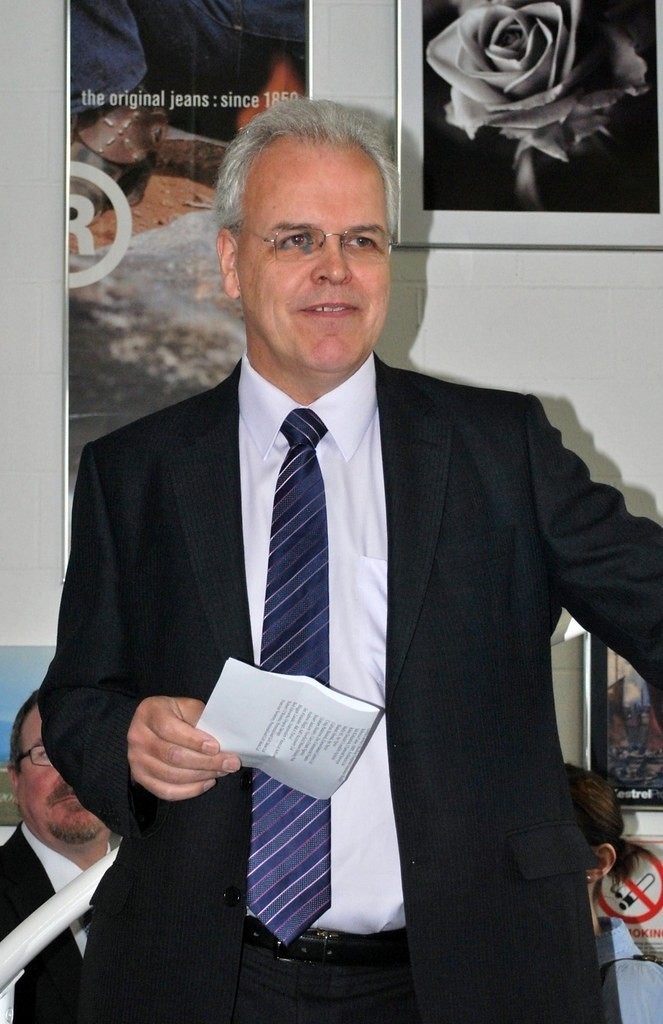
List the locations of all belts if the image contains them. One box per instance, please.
[247,915,413,970]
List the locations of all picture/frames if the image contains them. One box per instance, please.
[580,629,663,814]
[394,0,662,249]
[63,0,314,584]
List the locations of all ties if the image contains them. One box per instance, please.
[83,907,93,936]
[248,407,332,949]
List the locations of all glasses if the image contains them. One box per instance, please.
[239,224,399,262]
[15,744,53,767]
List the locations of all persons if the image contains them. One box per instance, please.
[36,96,663,1024]
[563,763,663,1023]
[0,686,113,1024]
